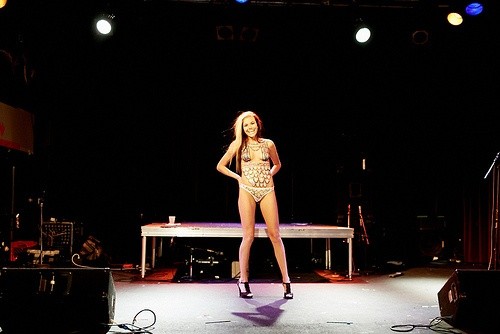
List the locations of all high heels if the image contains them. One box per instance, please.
[237,279,253,298]
[282,281,293,299]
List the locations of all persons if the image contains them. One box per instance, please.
[215,110,295,300]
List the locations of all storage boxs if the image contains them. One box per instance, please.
[183,237,239,282]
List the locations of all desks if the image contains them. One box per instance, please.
[140,221,355,281]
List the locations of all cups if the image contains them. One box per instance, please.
[169,216,175,224]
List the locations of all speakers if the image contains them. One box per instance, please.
[0,268,109,334]
[438,268,500,330]
